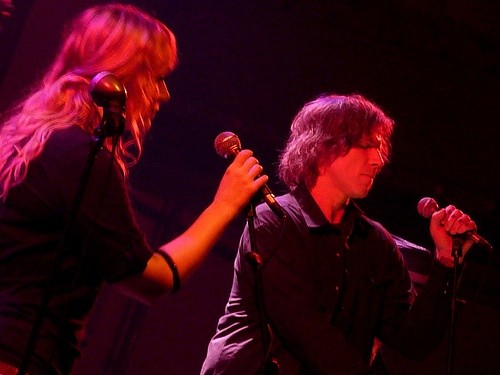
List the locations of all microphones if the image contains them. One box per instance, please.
[417,197,493,249]
[215,131,287,218]
[88,71,127,146]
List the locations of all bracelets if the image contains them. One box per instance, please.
[153,248,180,292]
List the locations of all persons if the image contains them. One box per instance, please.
[200,93,478,375]
[0,3,268,375]
